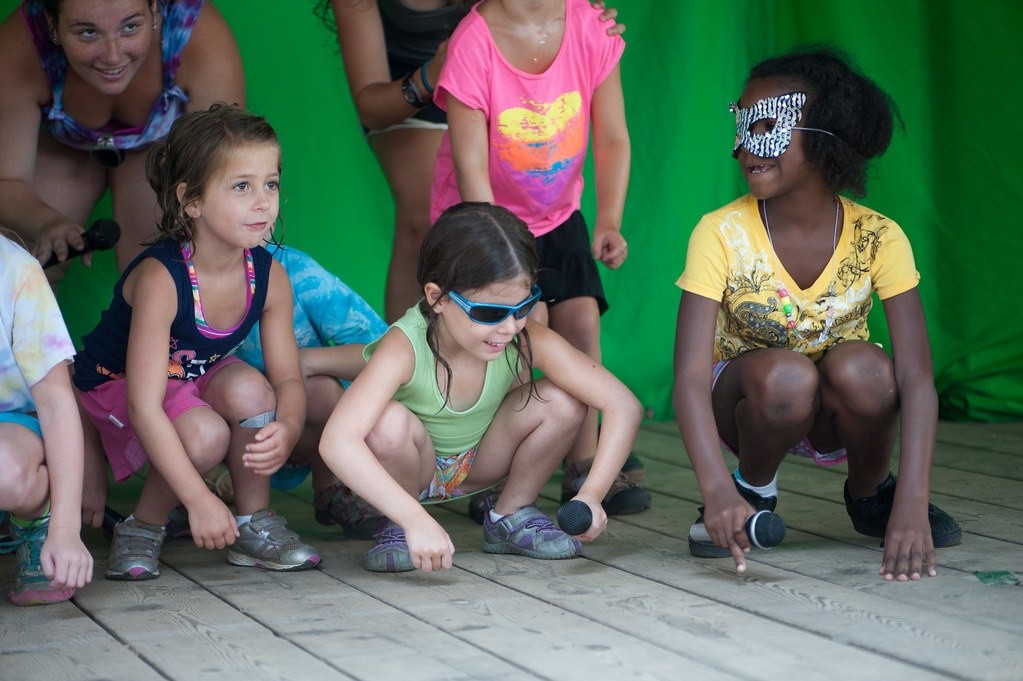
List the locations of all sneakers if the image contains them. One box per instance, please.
[104,520,164,580]
[363,526,415,572]
[8,519,74,606]
[165,507,191,540]
[468,488,499,522]
[561,457,650,514]
[314,486,389,540]
[226,508,322,573]
[484,506,584,559]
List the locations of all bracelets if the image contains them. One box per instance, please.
[420,60,435,92]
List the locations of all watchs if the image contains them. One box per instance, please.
[402,71,428,108]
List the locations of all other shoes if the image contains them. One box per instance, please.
[687,471,776,558]
[842,472,962,548]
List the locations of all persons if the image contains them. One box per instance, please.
[319,201,644,573]
[331,0,627,326]
[0,235,94,608]
[671,42,962,582]
[0,0,247,300]
[72,103,321,579]
[429,0,651,517]
[72,216,391,542]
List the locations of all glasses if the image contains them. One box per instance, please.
[89,132,128,168]
[442,285,542,324]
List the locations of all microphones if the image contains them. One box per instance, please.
[557,500,592,536]
[43,218,121,269]
[689,510,786,550]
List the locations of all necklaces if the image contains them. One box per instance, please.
[758,196,839,347]
